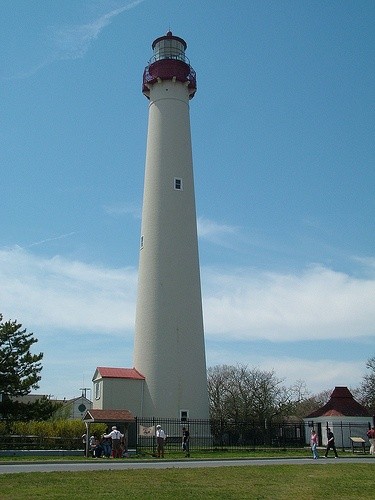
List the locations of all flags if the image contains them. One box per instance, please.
[138,425,155,436]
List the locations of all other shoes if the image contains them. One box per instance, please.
[324,457,327,458]
[185,456,187,457]
[335,457,337,459]
[188,454,190,457]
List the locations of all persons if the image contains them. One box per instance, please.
[310,430,319,459]
[156,425,166,458]
[82,426,129,459]
[367,426,375,455]
[182,428,190,457]
[323,428,338,459]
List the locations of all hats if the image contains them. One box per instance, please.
[157,424,161,428]
